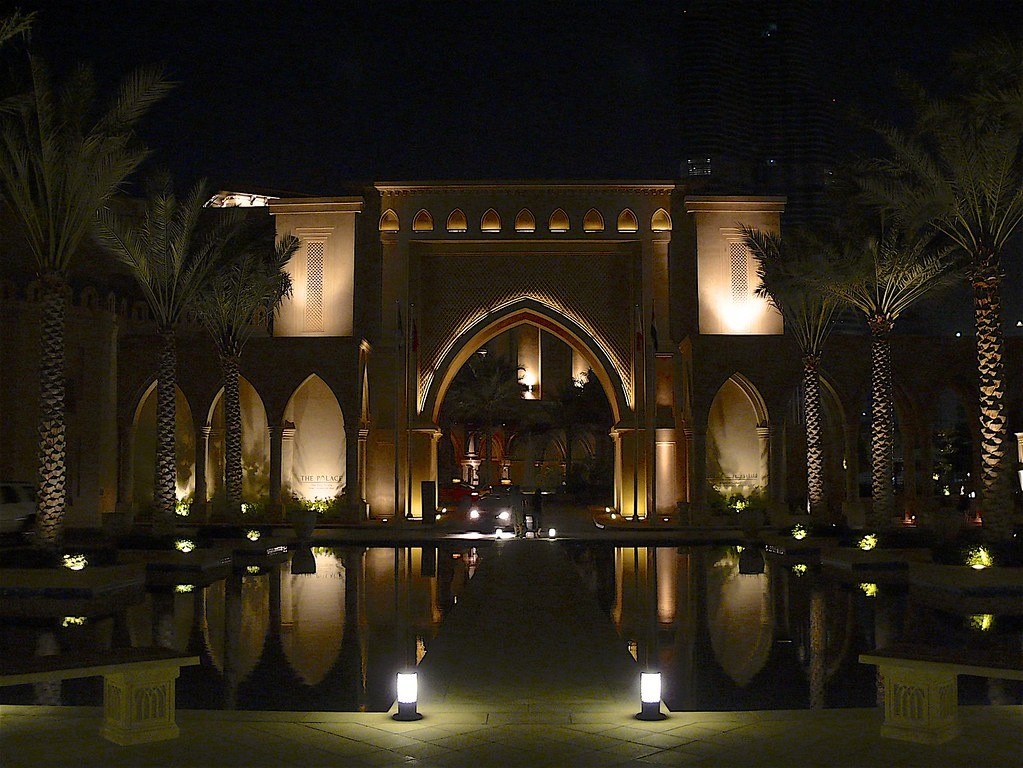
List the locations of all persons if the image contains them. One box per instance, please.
[507,485,528,539]
[532,488,544,538]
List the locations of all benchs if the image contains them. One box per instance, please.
[858,643,1023,745]
[0,645,200,743]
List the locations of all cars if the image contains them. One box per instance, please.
[438,478,527,527]
